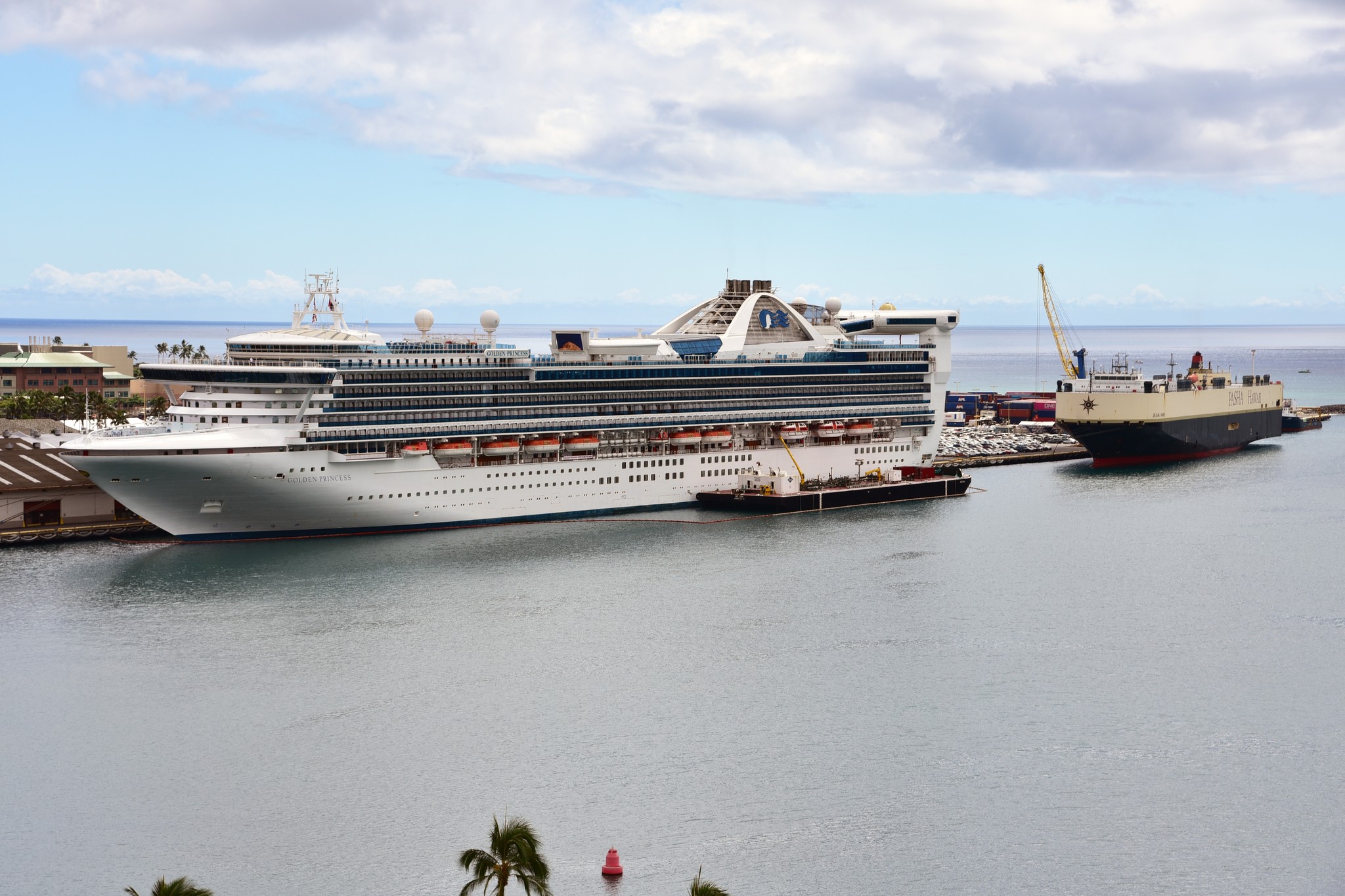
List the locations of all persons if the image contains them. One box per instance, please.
[250,357,253,366]
[133,513,137,519]
[601,358,604,365]
[171,403,174,406]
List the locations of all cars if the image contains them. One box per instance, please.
[937,424,1076,458]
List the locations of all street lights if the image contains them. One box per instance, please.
[972,389,980,426]
[1005,402,1012,438]
[990,386,998,402]
[1041,381,1047,397]
[954,382,961,394]
[140,385,149,422]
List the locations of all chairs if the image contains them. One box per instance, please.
[103,428,135,437]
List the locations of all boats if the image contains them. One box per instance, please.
[523,439,560,454]
[772,423,808,440]
[669,432,701,445]
[1298,369,1310,373]
[1133,359,1143,364]
[563,438,600,452]
[481,442,520,456]
[400,442,429,456]
[701,430,732,444]
[845,424,874,436]
[650,431,668,444]
[695,437,971,515]
[1034,263,1176,393]
[432,442,473,458]
[813,421,845,438]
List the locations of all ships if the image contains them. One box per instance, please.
[58,272,956,544]
[1055,349,1284,469]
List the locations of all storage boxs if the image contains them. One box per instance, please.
[893,466,935,478]
[944,390,1056,426]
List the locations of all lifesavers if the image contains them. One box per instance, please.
[327,379,332,383]
[135,431,138,435]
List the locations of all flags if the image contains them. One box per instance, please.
[312,297,317,322]
[331,302,333,311]
[328,298,332,311]
